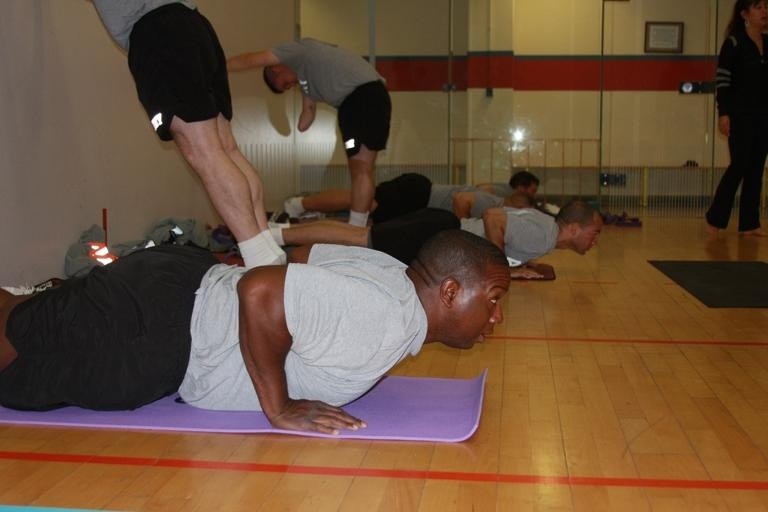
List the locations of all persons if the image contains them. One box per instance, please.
[477,169,541,200]
[284,172,537,228]
[267,198,603,280]
[705,2,767,240]
[2,227,510,434]
[226,38,392,227]
[88,0,288,269]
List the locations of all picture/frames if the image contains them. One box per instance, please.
[643,19,684,55]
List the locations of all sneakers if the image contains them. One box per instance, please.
[603,211,643,228]
